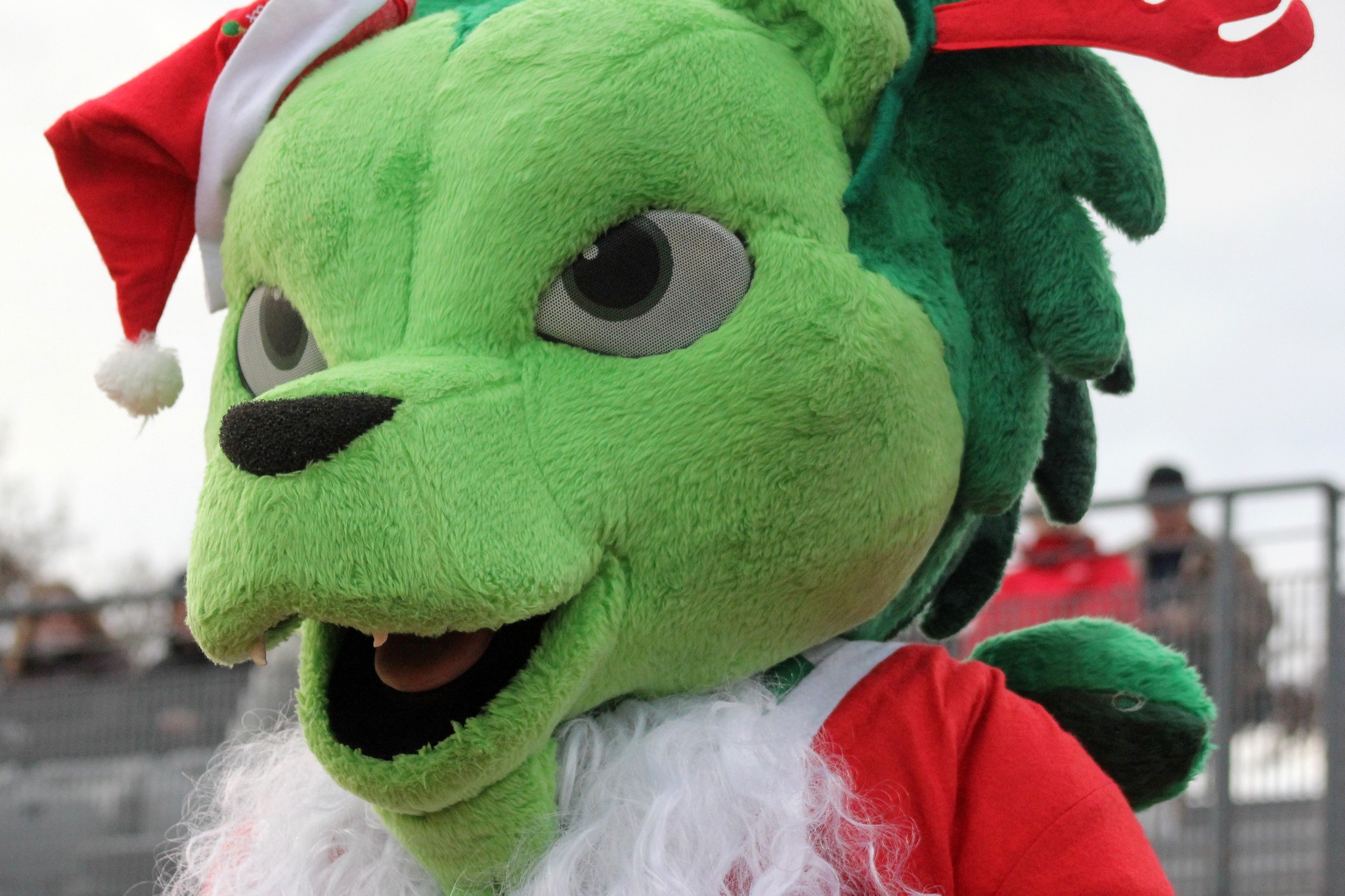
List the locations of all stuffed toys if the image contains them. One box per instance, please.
[48,1,1319,896]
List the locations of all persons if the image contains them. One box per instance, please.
[13,584,127,681]
[148,565,260,675]
[952,499,1144,668]
[1104,463,1278,747]
